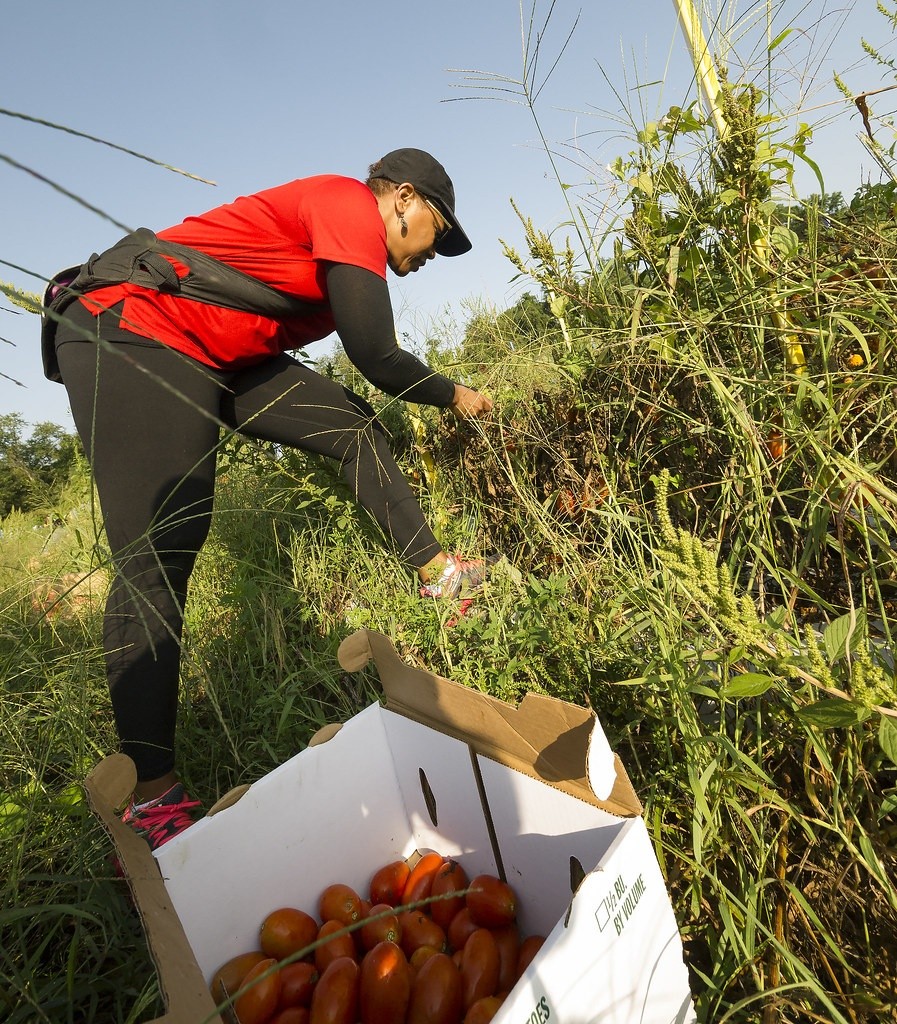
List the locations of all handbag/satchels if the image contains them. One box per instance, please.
[41,227,156,386]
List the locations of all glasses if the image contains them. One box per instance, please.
[396,185,453,250]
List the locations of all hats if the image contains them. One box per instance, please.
[369,148,472,257]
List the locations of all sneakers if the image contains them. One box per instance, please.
[122,782,202,853]
[420,552,502,601]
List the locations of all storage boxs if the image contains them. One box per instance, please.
[77,627,698,1024]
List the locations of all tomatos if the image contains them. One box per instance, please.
[211,853,548,1024]
[768,431,790,459]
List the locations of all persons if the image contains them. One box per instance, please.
[38,146,495,849]
